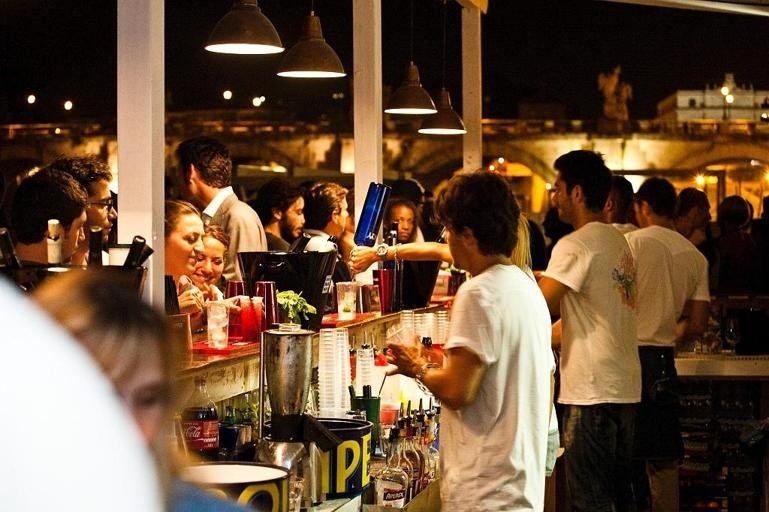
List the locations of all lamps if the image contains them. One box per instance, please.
[276,2,350,81]
[201,2,285,57]
[383,4,438,116]
[417,1,467,135]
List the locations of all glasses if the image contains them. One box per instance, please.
[436,227,448,244]
[91,197,113,209]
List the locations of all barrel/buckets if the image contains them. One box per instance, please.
[39,265,149,304]
[377,246,441,311]
[232,251,340,335]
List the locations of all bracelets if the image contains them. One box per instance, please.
[415,360,440,385]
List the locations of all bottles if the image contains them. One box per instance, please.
[123,233,156,267]
[354,181,393,247]
[348,385,357,399]
[374,396,439,508]
[383,221,399,268]
[223,403,253,453]
[362,383,372,398]
[291,232,312,252]
[45,217,68,269]
[326,233,336,242]
[180,374,221,462]
[87,222,103,265]
[1,227,21,267]
[437,225,447,245]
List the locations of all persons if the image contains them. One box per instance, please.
[351,209,562,512]
[620,176,711,509]
[1,138,769,351]
[382,165,554,512]
[24,270,260,512]
[536,150,644,511]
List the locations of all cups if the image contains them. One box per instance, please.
[335,281,358,321]
[253,280,279,327]
[398,310,449,345]
[357,349,376,396]
[351,396,380,453]
[204,301,230,349]
[316,327,353,420]
[224,280,249,298]
[237,294,264,341]
[377,268,402,313]
[166,312,193,355]
